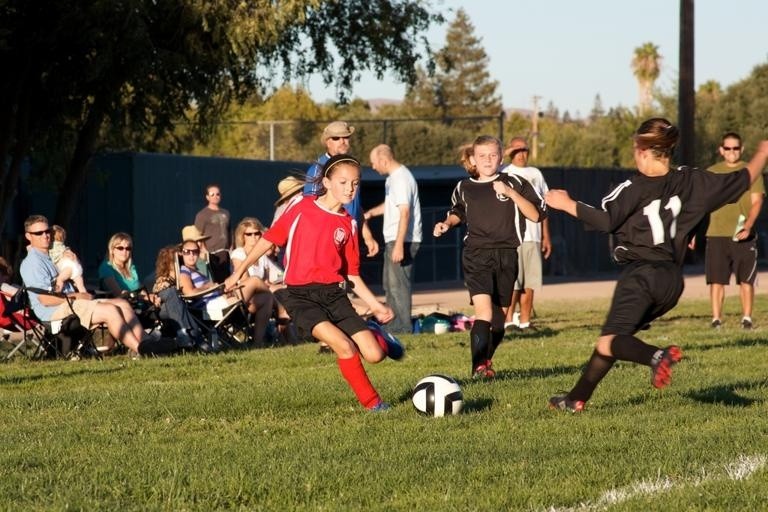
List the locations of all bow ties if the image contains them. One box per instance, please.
[713,320,720,327]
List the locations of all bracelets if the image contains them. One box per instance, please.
[367,209,375,217]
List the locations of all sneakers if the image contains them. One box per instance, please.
[549,397,585,414]
[650,346,683,389]
[475,360,496,381]
[367,320,403,360]
[741,320,752,329]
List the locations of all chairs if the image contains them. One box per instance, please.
[1,248,252,361]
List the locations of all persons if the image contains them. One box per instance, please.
[182,176,303,349]
[154,246,180,294]
[499,137,553,337]
[304,122,379,356]
[363,144,424,333]
[456,143,480,179]
[222,153,405,410]
[688,132,766,331]
[1,258,46,341]
[20,215,153,357]
[97,233,210,352]
[432,136,548,380]
[27,226,86,293]
[542,117,767,412]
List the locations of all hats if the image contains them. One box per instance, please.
[273,176,305,205]
[320,121,355,144]
[180,225,211,242]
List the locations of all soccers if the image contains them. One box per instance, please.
[412,375,464,417]
[422,312,452,335]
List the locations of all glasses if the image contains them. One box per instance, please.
[183,249,198,256]
[722,146,740,150]
[114,246,133,251]
[28,230,50,235]
[244,231,262,236]
